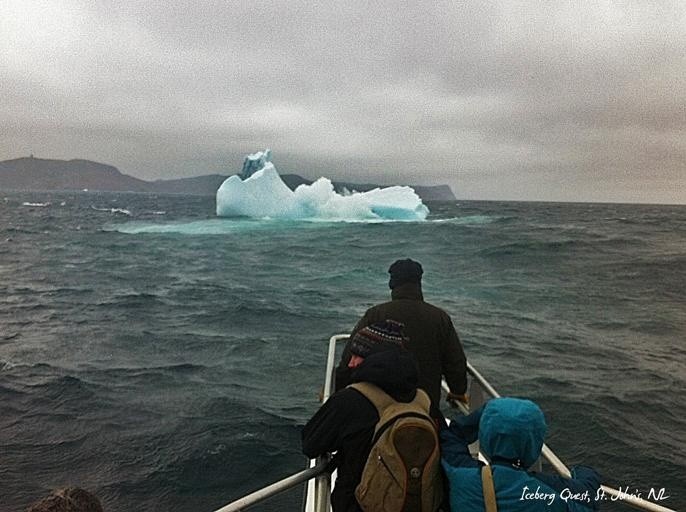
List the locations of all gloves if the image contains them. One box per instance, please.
[446,392,470,411]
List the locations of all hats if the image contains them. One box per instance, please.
[350,320,408,358]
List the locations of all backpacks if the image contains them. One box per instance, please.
[345,381,447,511]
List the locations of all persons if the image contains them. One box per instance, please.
[440,398,589,512]
[334,258,468,408]
[302,317,443,512]
